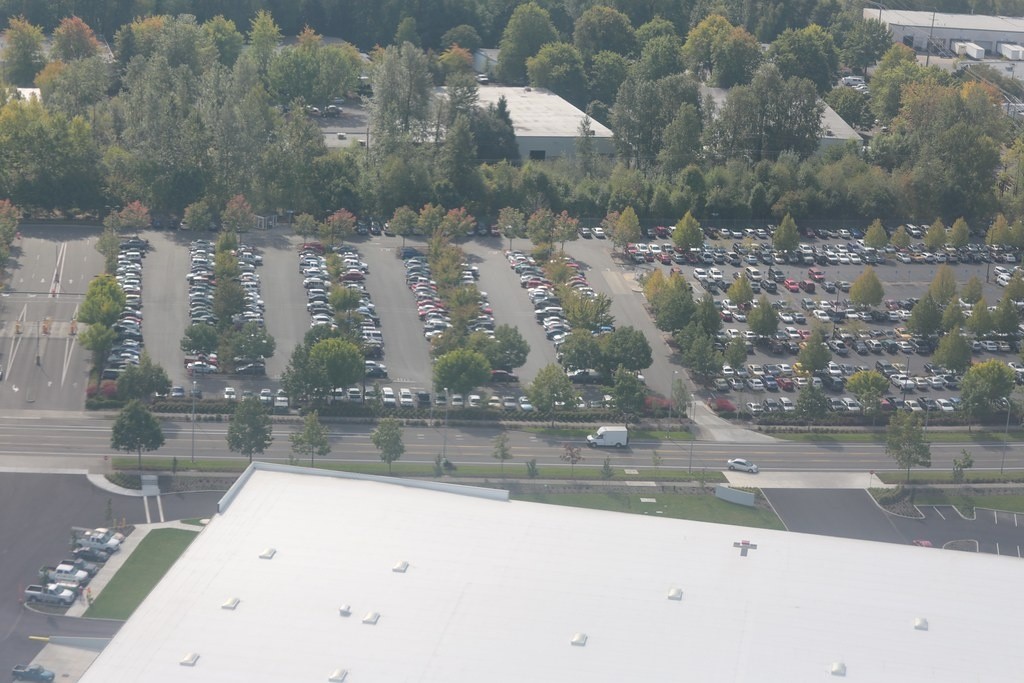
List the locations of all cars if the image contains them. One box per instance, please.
[307,104,343,118]
[101,233,149,378]
[505,249,645,385]
[728,458,759,473]
[297,242,388,379]
[183,239,265,374]
[912,539,933,548]
[842,76,870,96]
[153,218,988,239]
[475,74,488,84]
[625,240,1024,416]
[396,245,519,383]
[150,386,616,412]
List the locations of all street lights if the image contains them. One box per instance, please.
[186,380,196,474]
[666,370,696,474]
[443,388,448,462]
[1000,397,1011,474]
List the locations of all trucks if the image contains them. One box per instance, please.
[586,426,627,448]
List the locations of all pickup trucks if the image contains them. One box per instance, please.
[12,664,55,683]
[24,528,126,605]
